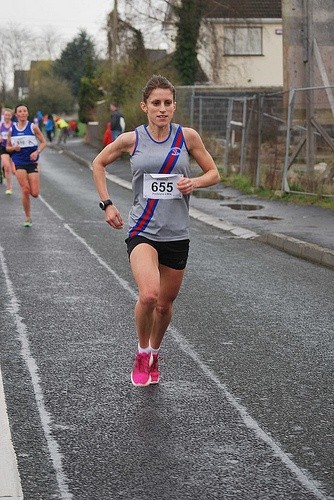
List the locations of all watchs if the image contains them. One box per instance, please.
[99,199,113,210]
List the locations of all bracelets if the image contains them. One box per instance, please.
[36,150,40,154]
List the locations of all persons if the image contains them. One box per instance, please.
[0,106,78,195]
[91,75,221,387]
[110,101,126,161]
[5,103,47,227]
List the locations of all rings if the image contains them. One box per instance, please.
[187,184,190,187]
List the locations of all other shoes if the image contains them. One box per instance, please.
[5,187,12,195]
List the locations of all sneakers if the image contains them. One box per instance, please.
[130,352,153,387]
[148,353,161,383]
[24,216,32,227]
